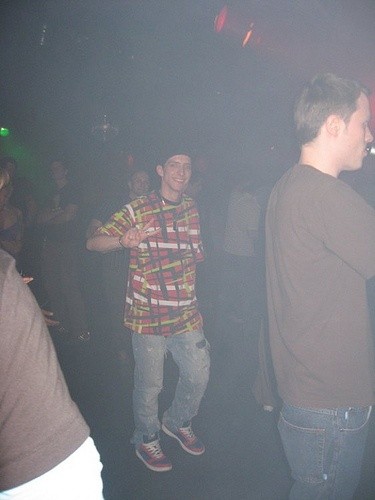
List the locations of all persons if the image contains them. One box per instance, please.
[84,145,212,473]
[1,246,104,499]
[261,68,375,500]
[1,141,281,417]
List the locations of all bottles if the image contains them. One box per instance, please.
[367,144,375,155]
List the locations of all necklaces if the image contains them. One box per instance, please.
[161,195,182,225]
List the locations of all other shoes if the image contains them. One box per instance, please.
[80,330,93,342]
[56,324,66,333]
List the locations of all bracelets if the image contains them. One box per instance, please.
[119,234,130,250]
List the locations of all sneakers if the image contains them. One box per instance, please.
[135,432,172,472]
[162,423,205,455]
